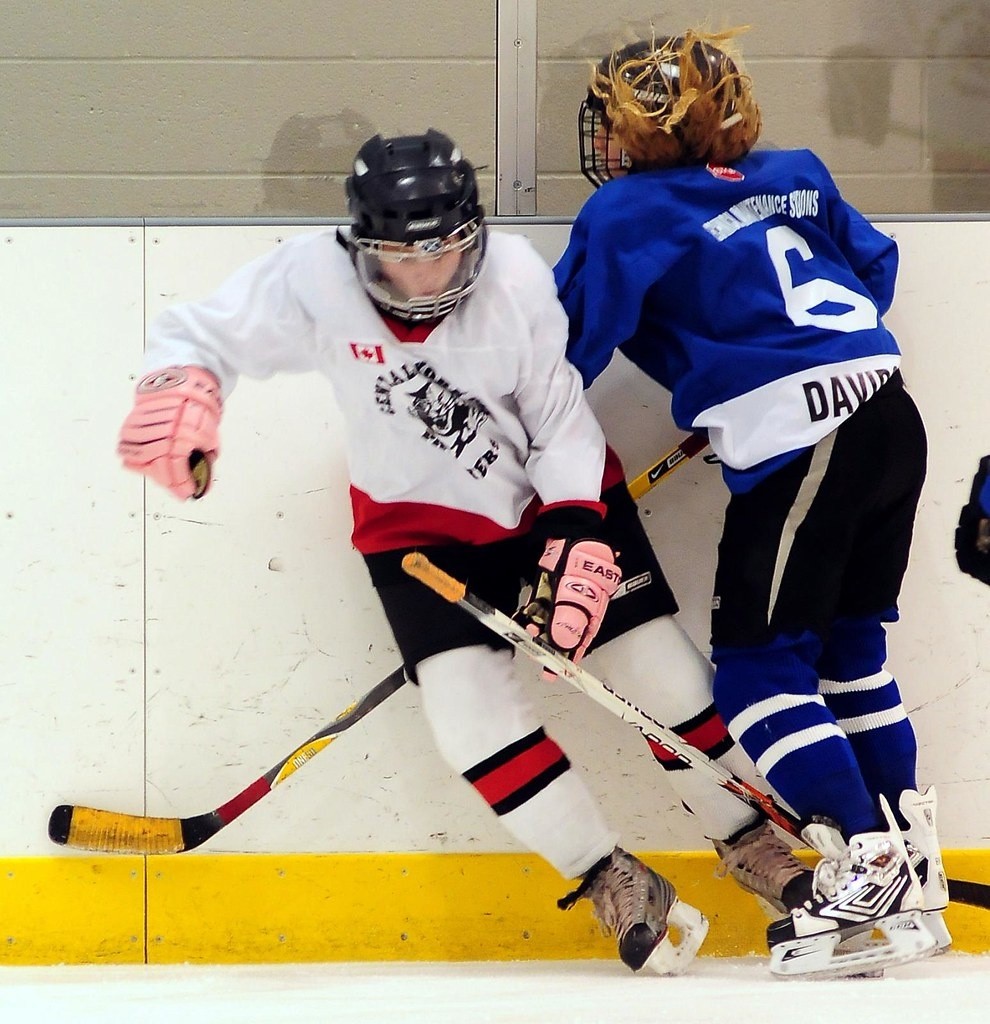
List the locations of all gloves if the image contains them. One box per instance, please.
[526,505,623,684]
[117,363,224,501]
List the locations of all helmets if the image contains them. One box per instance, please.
[577,35,742,190]
[336,128,488,323]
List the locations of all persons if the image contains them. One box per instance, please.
[550,32,953,978]
[116,126,820,976]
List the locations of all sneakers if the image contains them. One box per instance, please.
[766,793,938,979]
[557,847,709,976]
[898,784,954,949]
[712,822,815,922]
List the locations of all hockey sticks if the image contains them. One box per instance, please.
[47,426,711,856]
[400,551,990,913]
